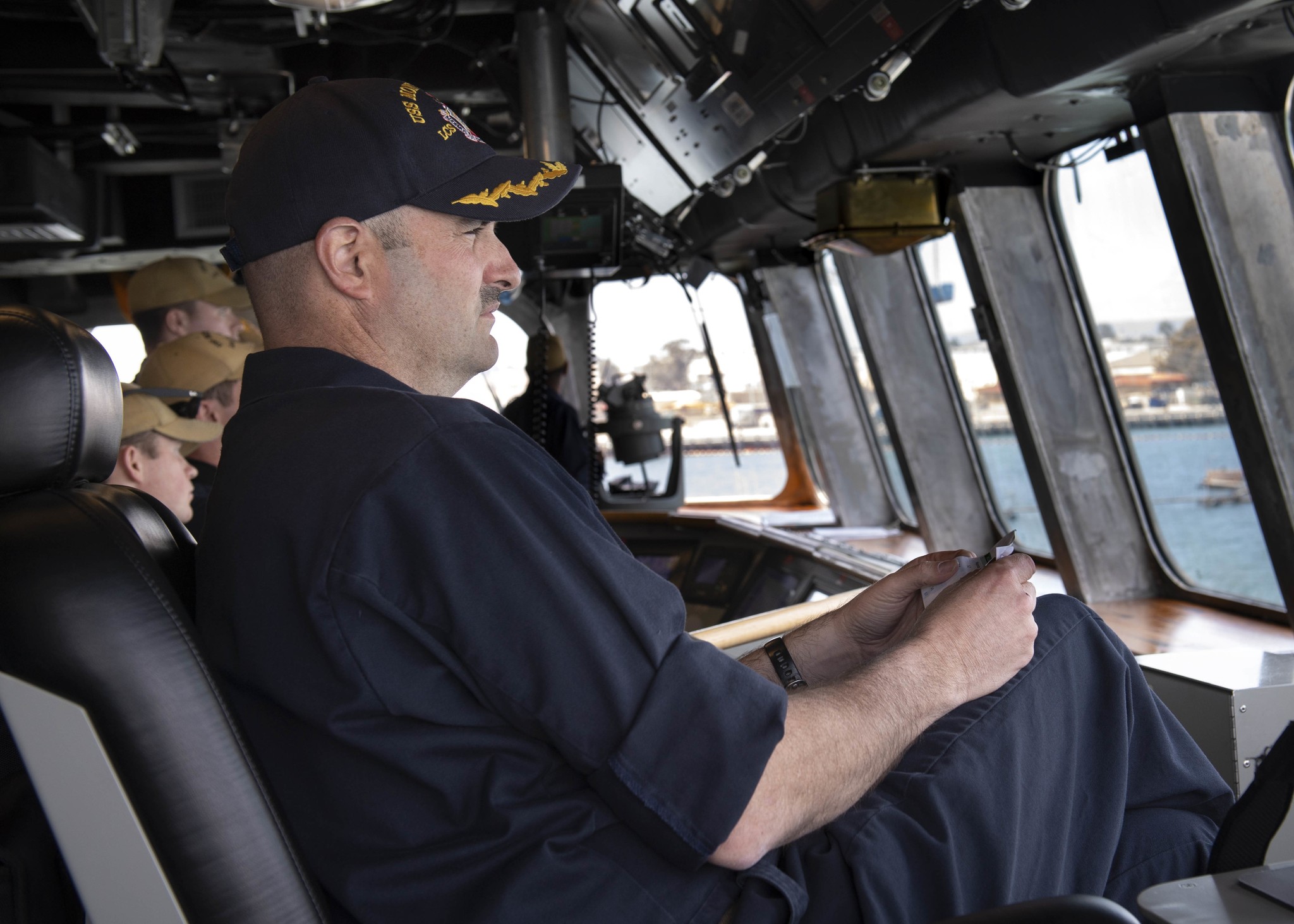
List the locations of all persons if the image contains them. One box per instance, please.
[102,254,265,524]
[500,335,585,478]
[196,75,1237,924]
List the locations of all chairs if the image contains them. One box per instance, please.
[1,304,1142,924]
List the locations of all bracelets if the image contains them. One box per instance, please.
[760,636,808,694]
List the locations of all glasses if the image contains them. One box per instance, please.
[123,386,203,420]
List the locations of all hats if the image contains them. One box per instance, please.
[200,284,253,311]
[238,321,262,344]
[220,76,583,282]
[128,257,232,312]
[122,382,224,457]
[131,331,255,404]
[528,335,566,372]
